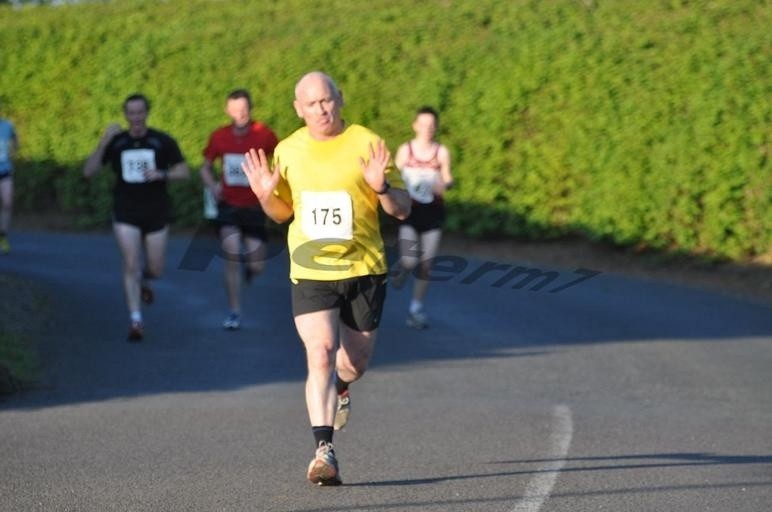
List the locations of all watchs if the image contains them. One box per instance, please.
[375,180,390,195]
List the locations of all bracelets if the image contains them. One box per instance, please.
[162,169,167,181]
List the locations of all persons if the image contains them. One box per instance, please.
[201,91,279,329]
[395,108,453,331]
[0,119,18,252]
[241,72,411,486]
[82,94,190,339]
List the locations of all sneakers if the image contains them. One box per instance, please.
[333,391,351,430]
[405,308,429,330]
[224,312,242,329]
[392,267,408,289]
[307,445,342,486]
[142,285,154,304]
[128,322,143,341]
[0,237,9,254]
